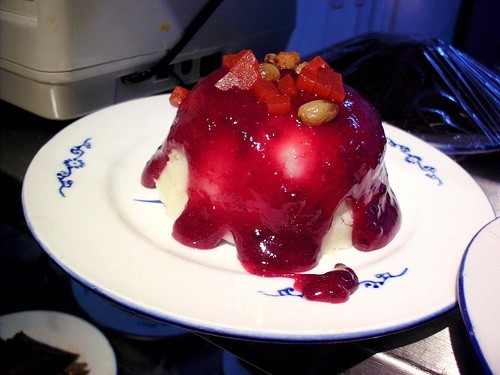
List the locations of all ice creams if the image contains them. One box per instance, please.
[142,48,401,303]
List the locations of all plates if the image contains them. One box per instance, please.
[70,278,194,338]
[21,94,499,347]
[0,311,120,375]
[458,212,499,375]
[422,121,498,163]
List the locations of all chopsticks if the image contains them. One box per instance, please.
[419,36,499,153]
